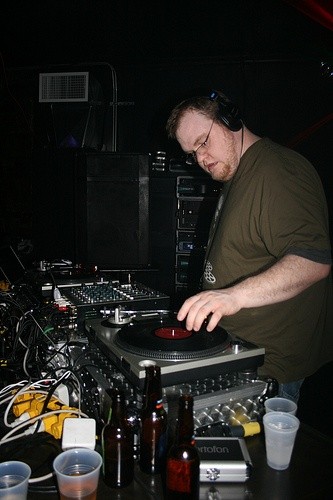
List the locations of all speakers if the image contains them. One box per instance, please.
[74,152,149,267]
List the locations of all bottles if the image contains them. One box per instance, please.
[159,396,200,500]
[195,421,261,438]
[136,366,170,490]
[100,392,135,490]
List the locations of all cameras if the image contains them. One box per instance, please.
[194,437,253,483]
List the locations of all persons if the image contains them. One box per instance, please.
[168,93,333,409]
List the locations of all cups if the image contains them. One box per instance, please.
[0,461,32,500]
[53,448,102,500]
[262,411,300,470]
[264,397,298,416]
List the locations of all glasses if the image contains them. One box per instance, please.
[187,114,217,164]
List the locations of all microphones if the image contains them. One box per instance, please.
[204,421,263,438]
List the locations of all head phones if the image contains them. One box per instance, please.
[181,87,242,131]
